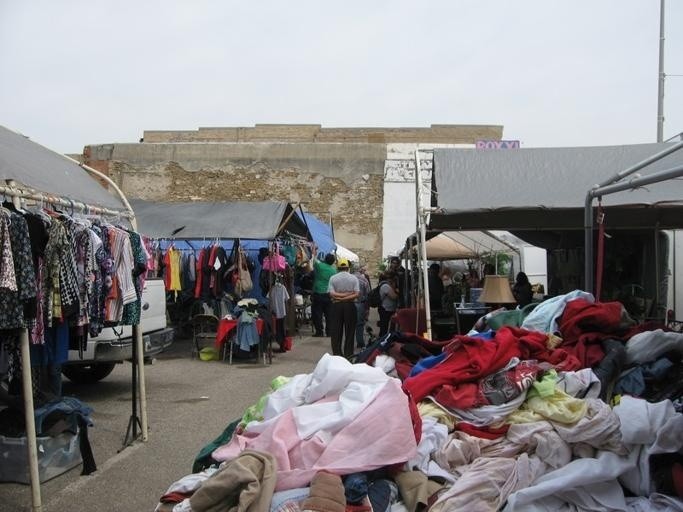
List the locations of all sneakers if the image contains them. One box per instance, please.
[357,341,367,348]
[311,329,332,338]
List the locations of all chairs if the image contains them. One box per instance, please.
[396,307,426,337]
[188,314,221,360]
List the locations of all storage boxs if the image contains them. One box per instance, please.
[0,425,81,486]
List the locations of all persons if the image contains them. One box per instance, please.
[476,264,496,288]
[375,267,400,339]
[389,256,412,312]
[426,263,444,314]
[356,264,370,290]
[440,269,482,315]
[512,271,534,308]
[349,262,370,350]
[325,258,360,359]
[310,241,339,338]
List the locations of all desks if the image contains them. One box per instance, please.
[219,318,264,365]
[293,302,314,341]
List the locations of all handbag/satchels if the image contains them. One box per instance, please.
[219,256,255,300]
[278,243,315,272]
[261,252,287,272]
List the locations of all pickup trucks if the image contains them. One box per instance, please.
[54,274,175,385]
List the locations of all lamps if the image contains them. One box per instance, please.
[475,272,516,309]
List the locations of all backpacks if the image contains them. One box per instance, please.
[367,281,389,308]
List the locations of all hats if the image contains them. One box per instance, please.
[335,257,351,269]
